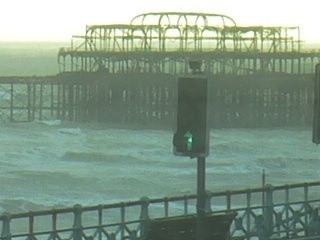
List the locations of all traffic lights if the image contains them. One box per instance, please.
[173,74,208,159]
